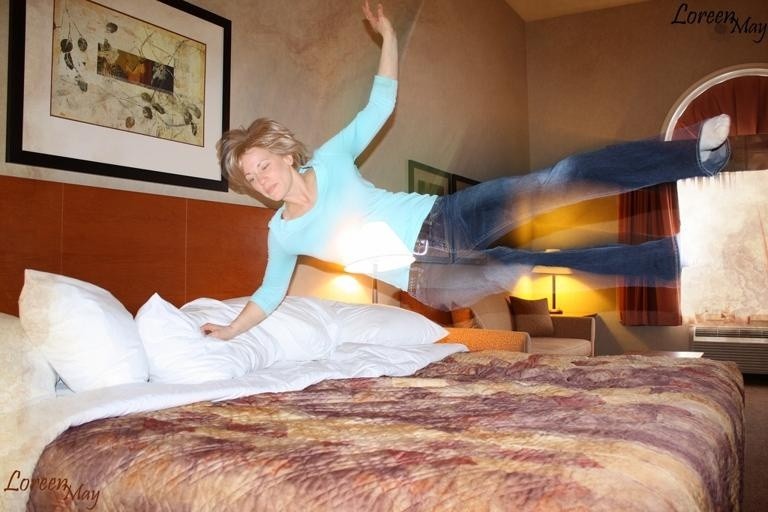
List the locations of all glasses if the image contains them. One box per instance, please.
[344,220,416,305]
[531,247,574,315]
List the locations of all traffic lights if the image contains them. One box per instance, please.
[18,267,148,393]
[134,291,283,384]
[221,295,346,364]
[0,312,60,412]
[509,295,555,338]
[308,297,451,347]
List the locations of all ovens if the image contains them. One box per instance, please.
[1,175,744,511]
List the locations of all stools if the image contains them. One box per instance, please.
[407,214,431,300]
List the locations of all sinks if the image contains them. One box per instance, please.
[398,287,596,357]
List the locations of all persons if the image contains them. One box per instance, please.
[199,0,730,345]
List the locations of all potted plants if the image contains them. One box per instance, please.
[454,175,487,266]
[6,0,232,193]
[407,159,453,266]
